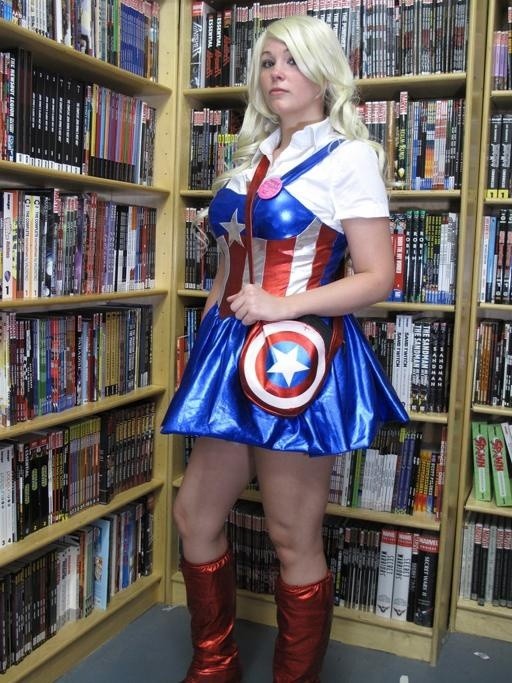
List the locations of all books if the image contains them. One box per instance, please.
[186,101,238,192]
[181,434,200,470]
[369,207,456,303]
[318,521,439,629]
[180,204,221,290]
[359,90,465,189]
[457,2,511,615]
[0,186,156,299]
[220,507,283,596]
[0,51,157,185]
[187,2,468,89]
[0,398,156,547]
[0,303,153,428]
[0,1,158,81]
[360,315,452,413]
[325,422,448,518]
[0,494,155,673]
[172,306,207,392]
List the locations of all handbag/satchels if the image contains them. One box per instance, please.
[239,315,346,417]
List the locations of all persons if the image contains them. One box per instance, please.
[160,14,410,682]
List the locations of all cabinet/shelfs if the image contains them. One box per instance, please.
[164,1,488,667]
[447,1,512,647]
[3,2,178,683]
